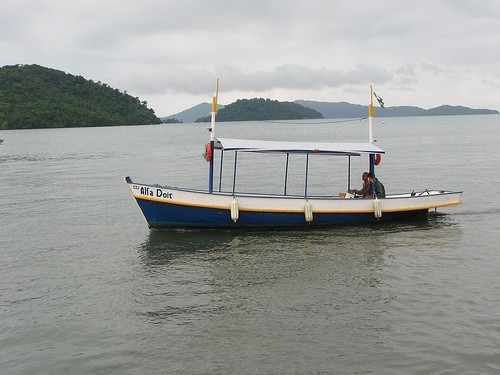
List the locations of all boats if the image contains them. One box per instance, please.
[125,79,463,230]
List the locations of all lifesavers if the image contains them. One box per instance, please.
[374,154,380,165]
[206,143,212,162]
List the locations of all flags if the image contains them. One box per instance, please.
[373,91,384,107]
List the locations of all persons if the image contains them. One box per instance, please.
[368,173,385,199]
[349,172,370,198]
